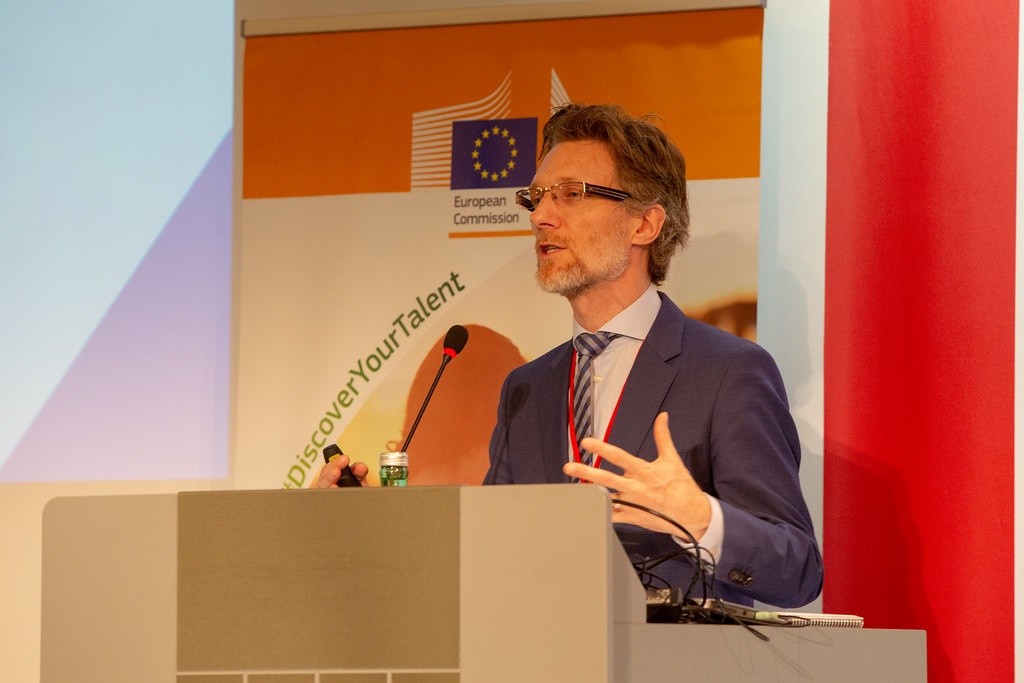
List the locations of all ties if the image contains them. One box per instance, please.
[569,330,619,483]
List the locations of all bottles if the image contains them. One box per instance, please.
[379,452,408,487]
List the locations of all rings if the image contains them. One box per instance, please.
[611,492,621,511]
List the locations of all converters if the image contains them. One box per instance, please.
[644,587,683,624]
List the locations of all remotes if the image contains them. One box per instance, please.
[323,444,361,487]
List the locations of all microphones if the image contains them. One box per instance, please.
[402,325,468,454]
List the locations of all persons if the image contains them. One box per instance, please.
[316,101,823,608]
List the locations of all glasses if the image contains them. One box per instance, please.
[514,180,631,212]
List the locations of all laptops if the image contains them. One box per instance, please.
[693,597,770,625]
